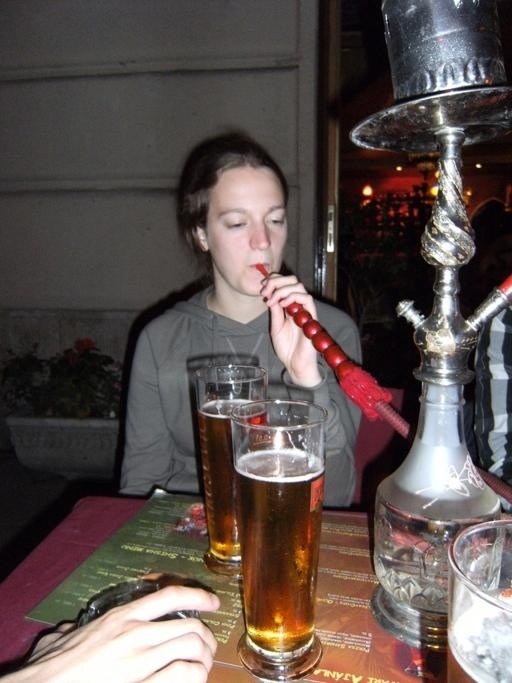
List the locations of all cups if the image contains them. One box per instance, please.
[445,519,512,683]
[229,400,329,682]
[191,365,269,572]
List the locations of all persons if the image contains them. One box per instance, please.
[119,132,363,512]
[0,585,221,683]
